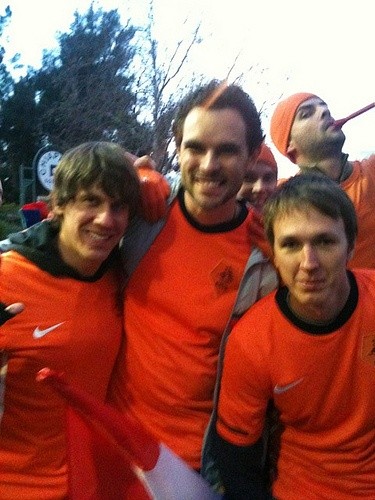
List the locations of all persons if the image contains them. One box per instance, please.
[214,171,375,499]
[271,91,374,277]
[0,140,171,500]
[123,79,280,500]
[235,144,279,212]
